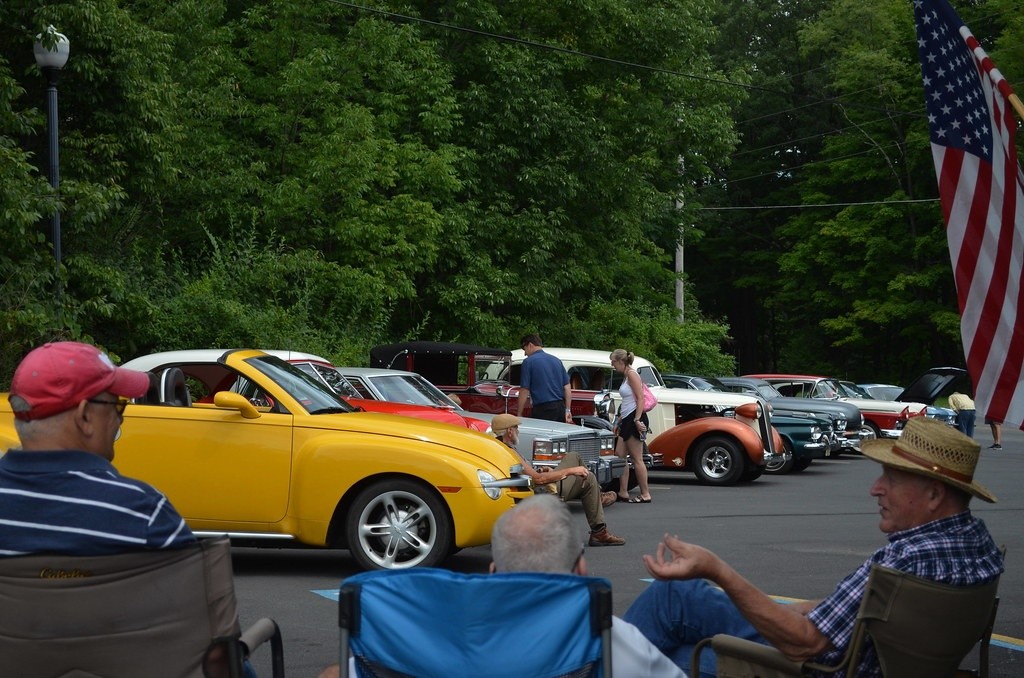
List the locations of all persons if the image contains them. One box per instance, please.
[489,413,626,547]
[516,334,574,423]
[317,494,687,678]
[610,349,652,503]
[620,415,1004,678]
[947,386,976,439]
[0,342,259,678]
[985,418,1003,451]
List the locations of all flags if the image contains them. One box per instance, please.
[912,0,1024,433]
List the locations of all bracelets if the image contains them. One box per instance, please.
[565,408,570,412]
[615,421,619,425]
[634,418,639,422]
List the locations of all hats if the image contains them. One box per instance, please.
[492,414,521,432]
[859,416,997,503]
[8,342,150,421]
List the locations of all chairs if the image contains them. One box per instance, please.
[339,567,615,678]
[0,535,284,678]
[690,546,1008,678]
[160,368,190,408]
[131,370,161,406]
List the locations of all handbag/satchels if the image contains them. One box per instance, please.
[642,383,657,412]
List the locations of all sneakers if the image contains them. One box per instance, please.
[589,526,625,545]
[600,491,617,506]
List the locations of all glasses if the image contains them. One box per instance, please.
[90,399,127,414]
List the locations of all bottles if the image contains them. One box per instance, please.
[640,422,647,441]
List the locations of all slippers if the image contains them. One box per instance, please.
[616,494,629,502]
[629,496,651,503]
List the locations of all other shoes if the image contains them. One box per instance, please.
[989,444,1002,450]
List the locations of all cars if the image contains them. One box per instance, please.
[509,347,792,484]
[223,351,493,438]
[740,367,977,451]
[334,366,626,484]
[0,350,535,572]
[370,342,616,432]
[659,373,875,477]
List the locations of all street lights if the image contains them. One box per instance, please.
[34,24,69,261]
